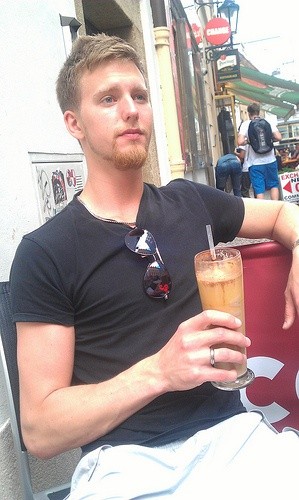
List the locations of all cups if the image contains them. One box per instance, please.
[193,247,255,392]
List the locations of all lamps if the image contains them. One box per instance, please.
[205,0,239,64]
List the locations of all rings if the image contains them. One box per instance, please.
[210,346,214,366]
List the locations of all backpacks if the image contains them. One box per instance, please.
[247,117,274,155]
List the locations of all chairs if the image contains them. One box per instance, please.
[1,281,70,500]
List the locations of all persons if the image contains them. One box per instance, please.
[9,33,299,500]
[233,103,299,200]
[216,153,241,197]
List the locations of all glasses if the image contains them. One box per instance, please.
[125,228,174,300]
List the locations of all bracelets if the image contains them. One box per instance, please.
[289,239,299,250]
